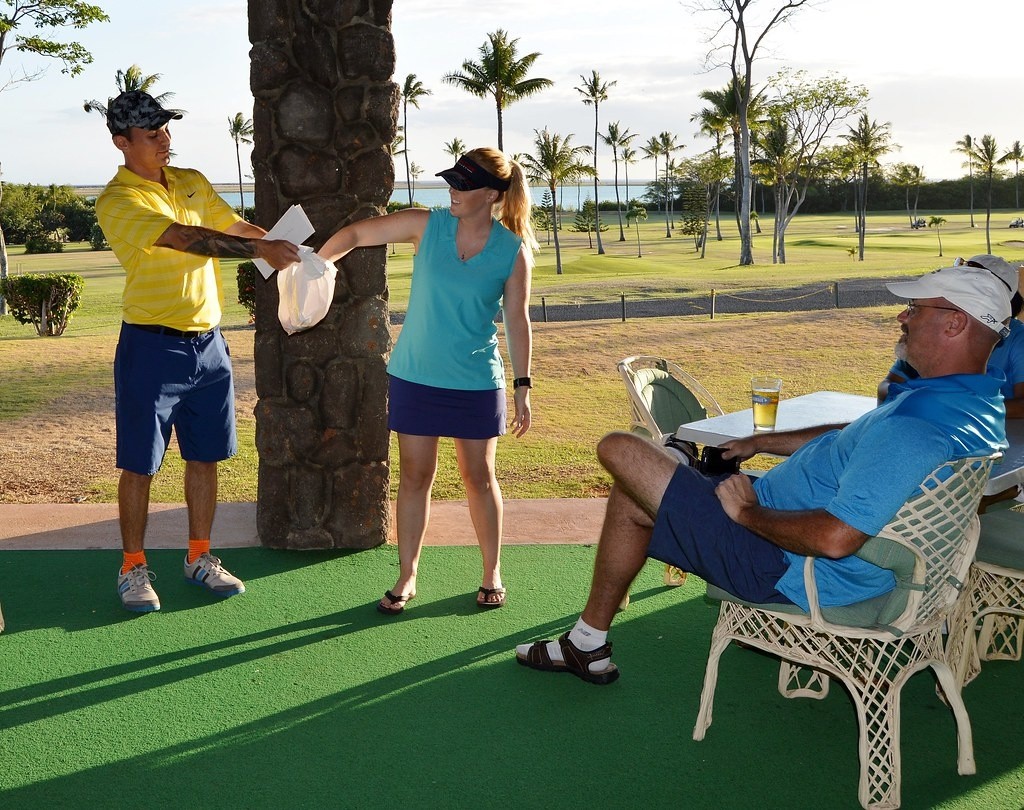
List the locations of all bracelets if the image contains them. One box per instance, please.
[514,377,533,389]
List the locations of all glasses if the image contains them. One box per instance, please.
[953,257,1013,291]
[906,300,958,317]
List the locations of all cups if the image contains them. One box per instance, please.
[751,376,781,431]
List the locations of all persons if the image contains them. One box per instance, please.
[94,90,301,611]
[317,147,531,615]
[515,266,1012,685]
[877,254,1024,514]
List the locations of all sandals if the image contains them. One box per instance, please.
[663,432,700,467]
[517,630,621,685]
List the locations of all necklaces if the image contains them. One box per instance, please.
[457,222,492,261]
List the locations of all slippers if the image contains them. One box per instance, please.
[378,589,414,615]
[477,586,506,608]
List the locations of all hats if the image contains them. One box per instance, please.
[969,253,1020,299]
[434,155,510,191]
[886,265,1015,339]
[107,90,183,132]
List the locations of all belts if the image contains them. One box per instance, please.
[122,320,218,337]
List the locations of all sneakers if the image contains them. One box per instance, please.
[184,550,246,594]
[118,565,161,611]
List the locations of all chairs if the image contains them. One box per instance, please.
[613,354,725,588]
[694,449,1006,810]
[968,463,1024,663]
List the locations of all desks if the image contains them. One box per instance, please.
[675,391,880,449]
